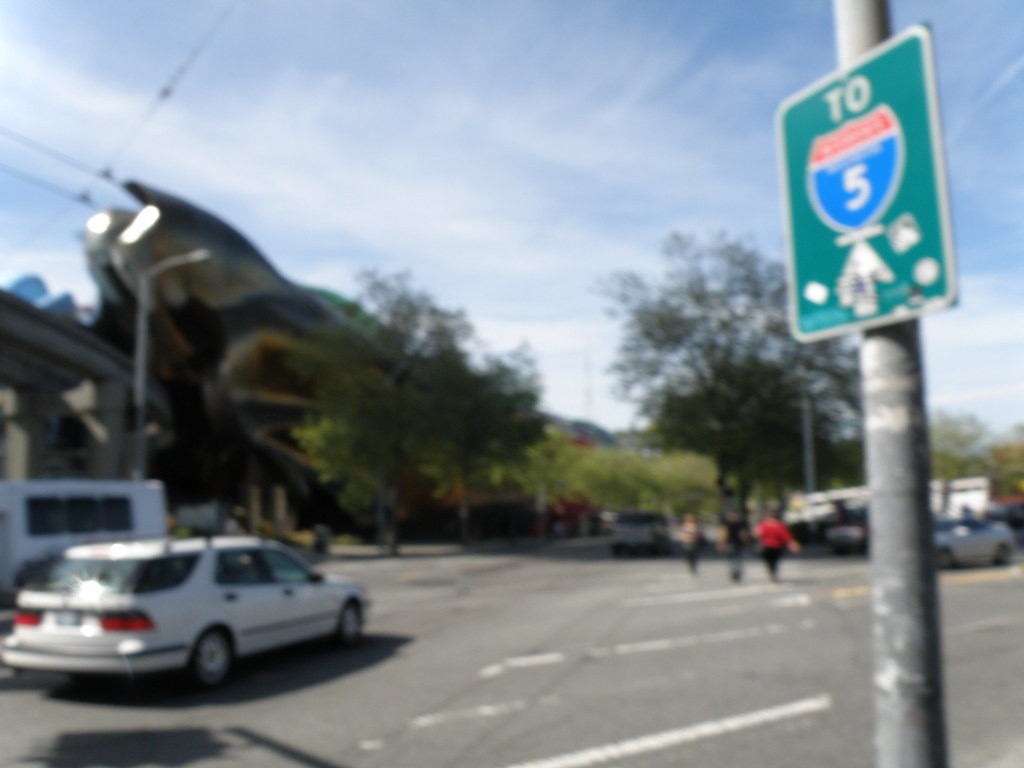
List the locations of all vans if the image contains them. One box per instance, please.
[0,466,170,605]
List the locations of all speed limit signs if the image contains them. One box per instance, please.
[776,22,957,348]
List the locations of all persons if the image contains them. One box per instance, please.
[718,505,751,581]
[680,511,708,575]
[755,507,800,583]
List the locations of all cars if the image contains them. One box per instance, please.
[1,520,377,698]
[932,510,1017,570]
